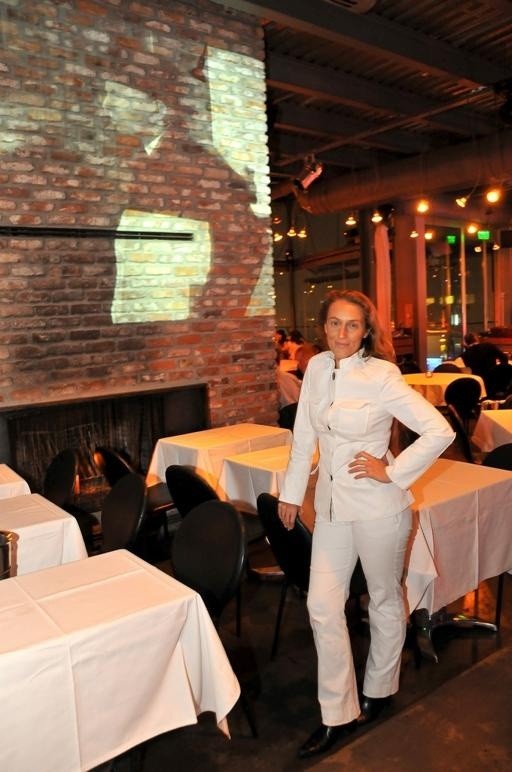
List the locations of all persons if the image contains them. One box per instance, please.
[460,333,508,375]
[276,287,459,760]
[276,329,320,411]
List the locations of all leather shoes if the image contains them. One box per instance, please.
[358,693,394,726]
[298,719,359,759]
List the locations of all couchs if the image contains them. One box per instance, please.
[0,382,208,549]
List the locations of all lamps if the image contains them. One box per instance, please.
[273,153,480,243]
